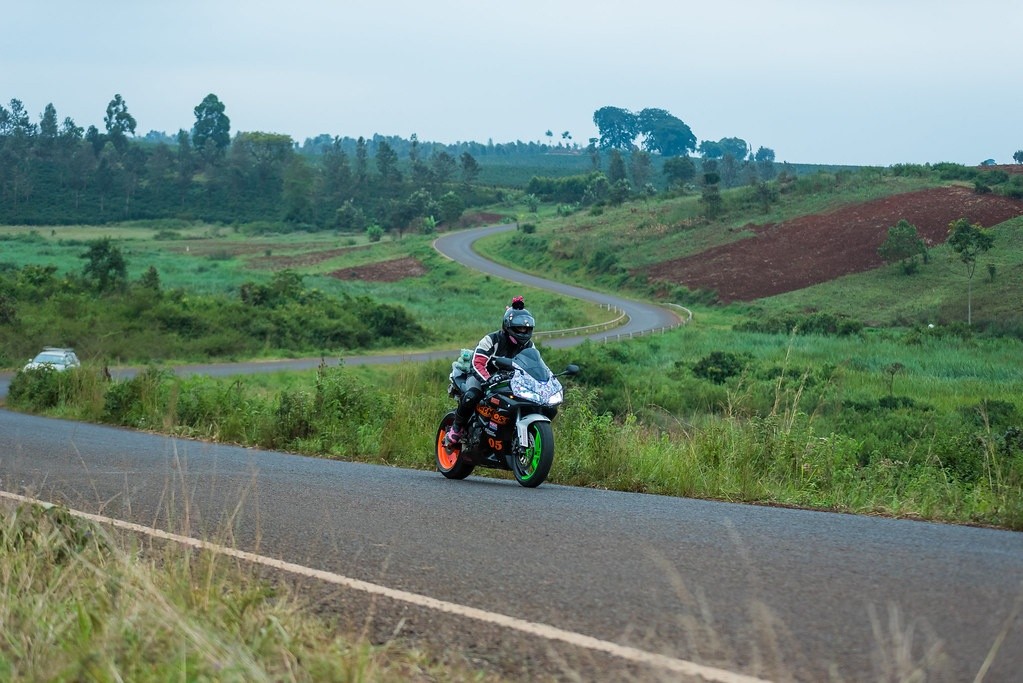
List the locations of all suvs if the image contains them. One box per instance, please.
[23,343,81,375]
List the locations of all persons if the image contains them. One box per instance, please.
[447,297,537,444]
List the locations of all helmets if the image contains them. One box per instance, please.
[502,306,535,346]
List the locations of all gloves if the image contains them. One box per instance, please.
[488,375,501,384]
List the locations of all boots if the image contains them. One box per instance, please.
[447,410,465,443]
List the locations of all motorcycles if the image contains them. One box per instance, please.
[435,347,579,488]
